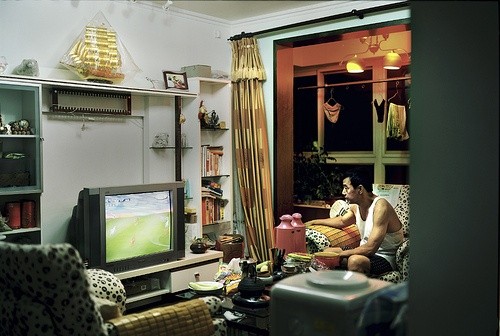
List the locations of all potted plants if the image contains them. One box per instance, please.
[294,140,345,206]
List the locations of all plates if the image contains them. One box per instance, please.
[307,270,368,288]
[188,281,224,293]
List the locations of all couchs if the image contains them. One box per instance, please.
[1,239,228,336]
[304,183,408,282]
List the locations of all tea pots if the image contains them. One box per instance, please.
[238,264,265,297]
[190,239,210,253]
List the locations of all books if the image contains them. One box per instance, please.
[203,147,224,177]
[202,181,224,224]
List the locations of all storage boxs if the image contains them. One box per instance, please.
[180,65,211,77]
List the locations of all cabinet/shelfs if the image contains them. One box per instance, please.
[144,77,234,250]
[108,247,224,311]
[1,77,42,246]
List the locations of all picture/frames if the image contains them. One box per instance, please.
[163,72,188,88]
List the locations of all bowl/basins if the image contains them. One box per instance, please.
[314,252,340,268]
[284,265,295,271]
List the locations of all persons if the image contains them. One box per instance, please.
[304,173,404,275]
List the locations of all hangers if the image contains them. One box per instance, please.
[372,88,386,106]
[408,97,411,111]
[323,91,345,112]
[387,82,406,102]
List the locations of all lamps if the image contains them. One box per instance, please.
[339,35,410,73]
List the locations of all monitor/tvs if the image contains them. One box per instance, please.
[70,181,185,274]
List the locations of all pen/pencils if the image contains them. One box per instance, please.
[271,248,278,258]
[279,250,281,257]
[282,249,285,258]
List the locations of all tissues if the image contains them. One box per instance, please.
[214,257,242,293]
